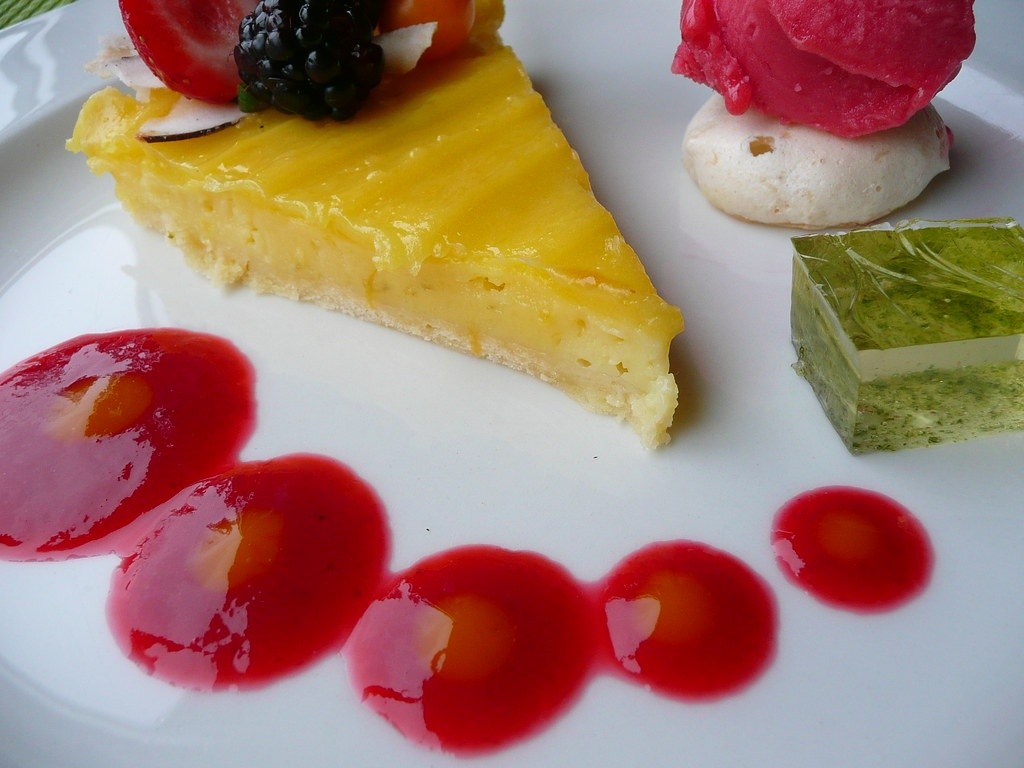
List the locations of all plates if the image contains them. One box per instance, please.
[0,0,1024,768]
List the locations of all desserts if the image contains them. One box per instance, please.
[673,0,978,229]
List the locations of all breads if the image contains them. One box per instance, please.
[65,0,683,452]
